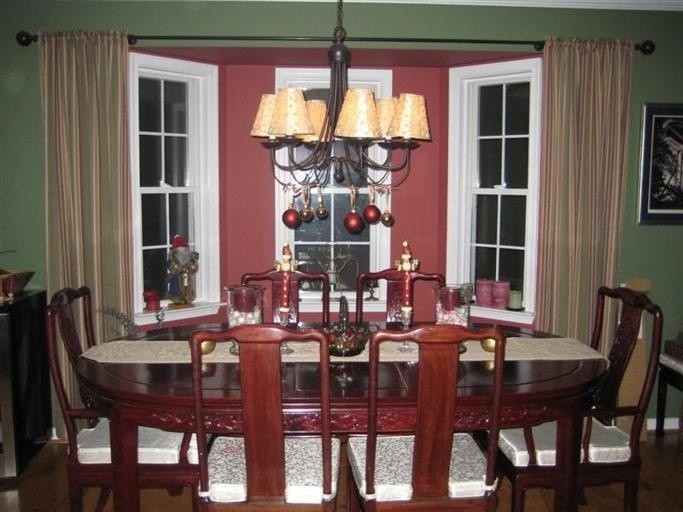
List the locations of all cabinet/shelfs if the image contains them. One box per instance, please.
[0,289,52,477]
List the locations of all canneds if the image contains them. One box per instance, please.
[476,278,522,309]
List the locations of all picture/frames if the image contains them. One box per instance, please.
[636,101,683,225]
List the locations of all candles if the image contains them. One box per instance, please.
[273,241,298,328]
[395,240,420,330]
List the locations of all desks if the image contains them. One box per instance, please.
[76,322,608,511]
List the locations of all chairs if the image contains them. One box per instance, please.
[190,324,341,512]
[239,271,332,333]
[472,285,665,511]
[346,323,504,512]
[356,269,446,331]
[44,285,210,510]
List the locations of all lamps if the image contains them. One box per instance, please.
[250,0,432,235]
